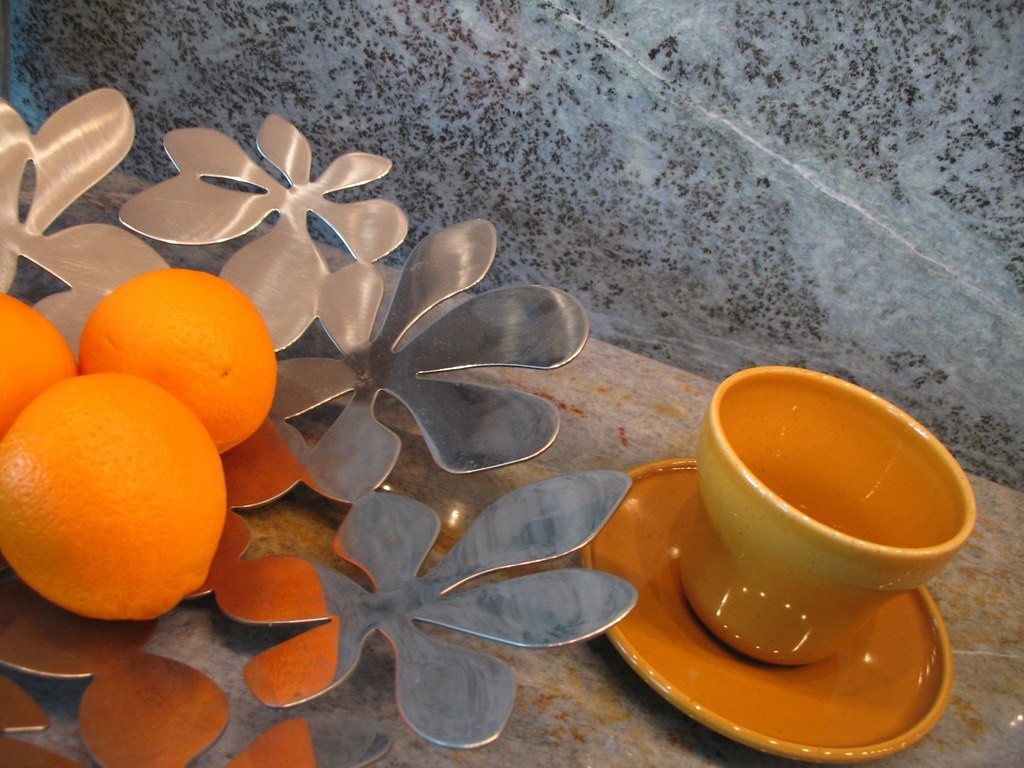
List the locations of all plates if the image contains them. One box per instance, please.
[583,458,956,765]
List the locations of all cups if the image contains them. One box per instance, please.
[679,366,977,665]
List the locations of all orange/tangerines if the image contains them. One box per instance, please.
[0,267,276,620]
